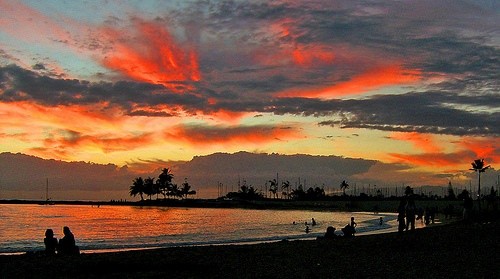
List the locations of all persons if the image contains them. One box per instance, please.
[305,222,307,226]
[416,205,468,225]
[57,227,75,257]
[398,199,407,233]
[312,218,316,226]
[325,227,338,243]
[44,229,58,258]
[379,216,383,226]
[351,216,357,236]
[305,226,311,233]
[293,221,295,224]
[407,195,418,231]
